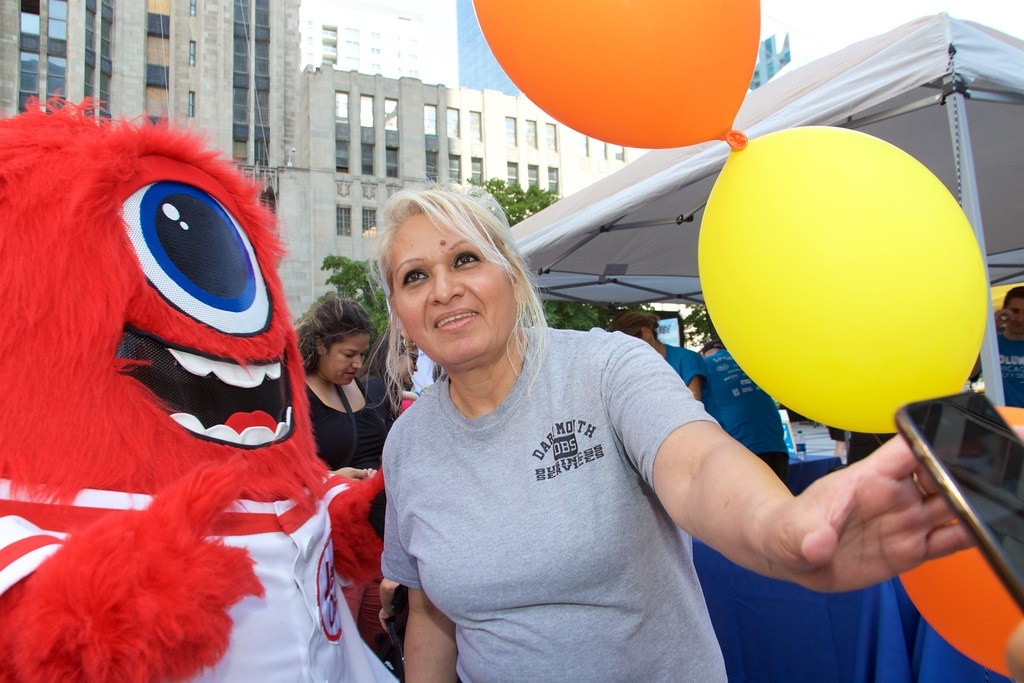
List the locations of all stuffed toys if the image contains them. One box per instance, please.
[0,92,400,683]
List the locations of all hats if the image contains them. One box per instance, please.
[701,340,728,352]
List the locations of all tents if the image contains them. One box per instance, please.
[509,11,1024,407]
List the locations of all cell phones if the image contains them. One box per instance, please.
[895,392,1024,617]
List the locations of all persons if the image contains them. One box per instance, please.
[371,179,978,683]
[293,285,1024,683]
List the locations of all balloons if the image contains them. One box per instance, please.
[470,0,1024,682]
